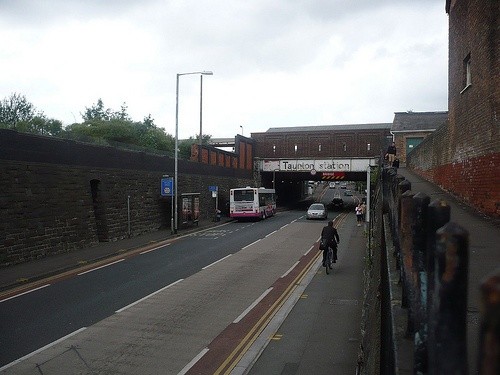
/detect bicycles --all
[320,238,339,275]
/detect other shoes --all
[322,261,326,267]
[357,224,361,226]
[332,260,336,263]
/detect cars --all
[340,184,346,189]
[305,202,328,221]
[329,182,335,189]
[332,197,343,207]
[344,190,353,196]
[335,181,340,184]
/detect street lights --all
[273,169,280,189]
[171,69,213,235]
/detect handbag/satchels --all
[319,239,329,250]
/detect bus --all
[227,186,277,223]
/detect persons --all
[361,202,366,222]
[355,204,362,224]
[387,142,396,167]
[216,210,221,222]
[321,221,339,267]
